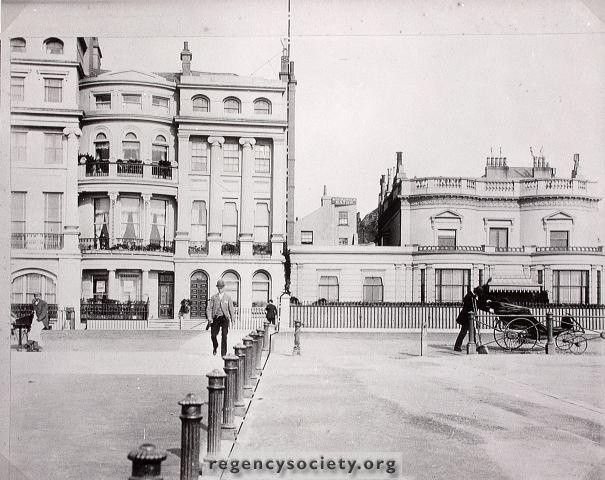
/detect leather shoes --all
[212,348,217,356]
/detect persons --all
[265,299,277,331]
[453,285,495,352]
[32,292,49,330]
[205,280,235,357]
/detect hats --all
[215,280,225,287]
